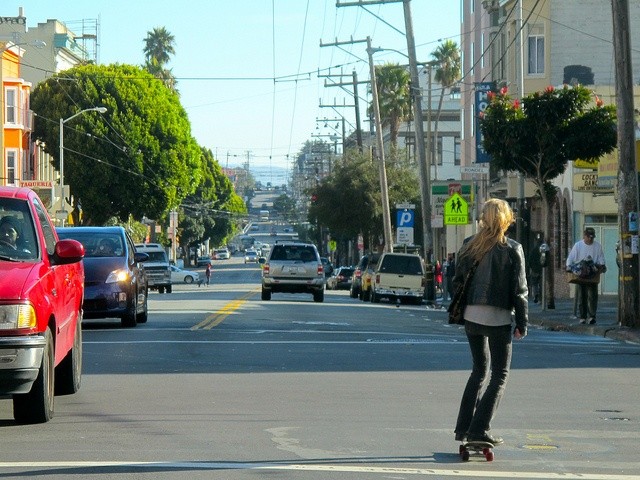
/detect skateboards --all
[459,437,494,461]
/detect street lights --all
[0,40,47,183]
[60,107,107,227]
[366,47,433,248]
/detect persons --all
[528,240,542,304]
[566,227,607,326]
[453,199,528,446]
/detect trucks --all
[259,210,269,221]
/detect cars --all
[282,227,294,232]
[262,204,268,209]
[261,243,325,302]
[326,268,339,289]
[360,253,381,301]
[226,244,238,255]
[321,257,333,276]
[270,229,278,237]
[332,267,355,290]
[350,256,369,298]
[256,186,280,191]
[250,224,259,231]
[54,226,149,327]
[244,251,258,264]
[248,240,277,258]
[216,249,230,260]
[197,256,211,266]
[171,265,200,284]
[370,253,426,305]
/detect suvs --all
[136,247,172,293]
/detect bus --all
[239,235,252,252]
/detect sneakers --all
[580,318,587,325]
[588,317,595,325]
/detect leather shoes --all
[454,430,469,440]
[466,431,504,446]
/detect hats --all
[584,227,596,238]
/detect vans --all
[134,243,161,248]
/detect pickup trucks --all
[0,184,85,423]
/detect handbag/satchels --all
[447,258,479,326]
